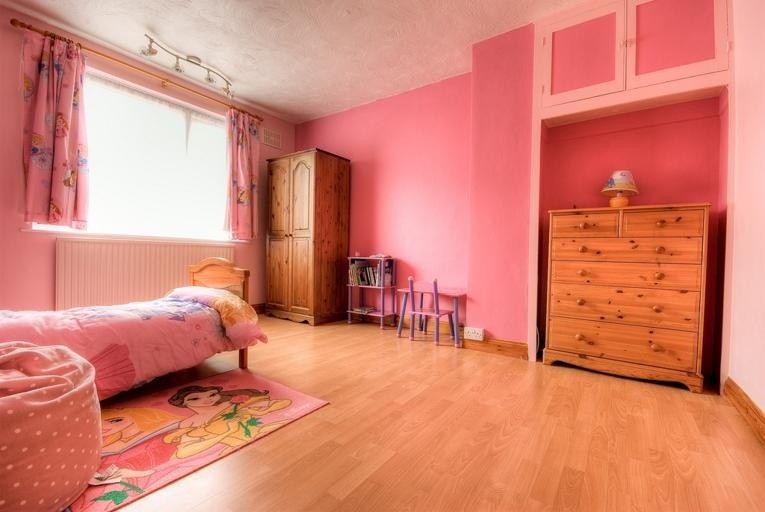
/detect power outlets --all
[463,327,485,342]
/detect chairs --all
[408,275,454,346]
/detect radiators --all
[55,236,235,310]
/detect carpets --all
[59,368,329,512]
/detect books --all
[352,306,374,315]
[349,260,393,286]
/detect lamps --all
[144,34,234,100]
[601,169,639,208]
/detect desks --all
[396,288,467,348]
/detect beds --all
[0,256,268,402]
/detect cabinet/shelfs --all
[543,201,712,394]
[540,0,730,130]
[346,256,398,331]
[265,147,350,326]
